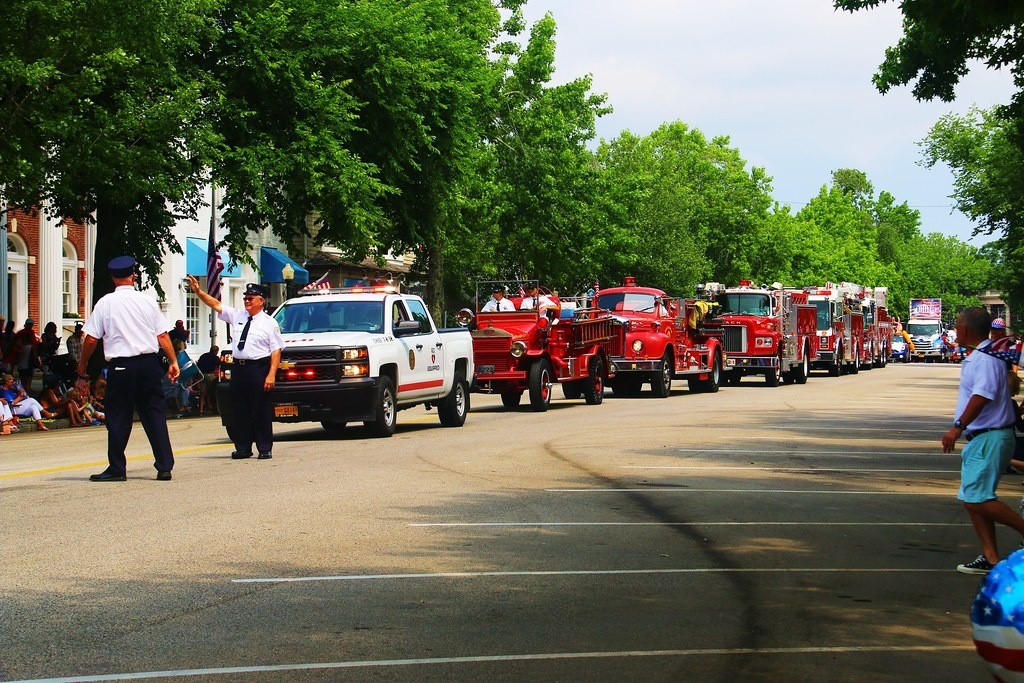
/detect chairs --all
[308,310,332,331]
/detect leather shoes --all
[157,472,171,479]
[258,451,273,459]
[90,471,126,481]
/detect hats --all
[176,320,182,326]
[25,318,34,324]
[491,285,506,292]
[528,282,537,289]
[244,283,265,296]
[108,256,135,275]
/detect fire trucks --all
[453,277,894,412]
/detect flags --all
[978,335,1024,367]
[206,221,225,302]
[303,277,332,289]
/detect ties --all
[497,302,500,311]
[533,298,536,306]
[238,315,253,350]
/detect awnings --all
[186,237,241,277]
[261,248,309,284]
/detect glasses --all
[243,297,254,301]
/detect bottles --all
[17,387,27,397]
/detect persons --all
[520,283,558,317]
[481,286,516,312]
[0,315,106,434]
[942,307,1024,574]
[189,275,286,460]
[78,257,180,482]
[162,320,220,418]
[990,318,1018,373]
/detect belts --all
[965,426,1011,441]
[233,358,264,366]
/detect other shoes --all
[39,426,49,431]
[46,412,58,420]
[71,423,81,427]
[82,423,91,426]
[12,426,20,431]
[1008,465,1024,475]
[173,412,184,418]
[199,411,206,417]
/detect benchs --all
[509,296,561,326]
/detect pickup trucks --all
[268,286,475,438]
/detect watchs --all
[954,420,966,430]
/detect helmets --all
[991,317,1006,329]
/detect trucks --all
[907,318,946,363]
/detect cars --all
[891,336,912,363]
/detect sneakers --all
[91,421,101,425]
[957,554,999,574]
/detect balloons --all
[969,548,1024,683]
